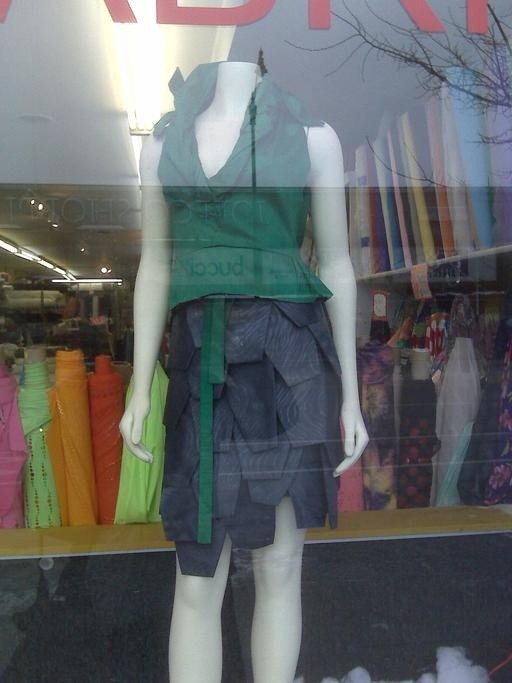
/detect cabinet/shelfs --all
[317,59,510,511]
